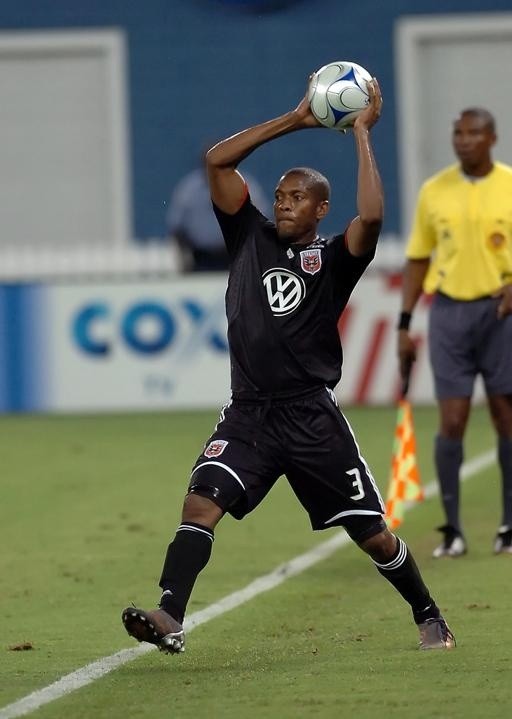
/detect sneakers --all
[415,613,458,653]
[491,523,512,556]
[431,521,469,560]
[120,602,187,657]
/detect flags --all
[380,399,425,532]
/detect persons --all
[122,62,452,660]
[394,106,512,556]
[163,136,271,271]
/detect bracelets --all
[396,311,411,330]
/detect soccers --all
[309,61,375,129]
